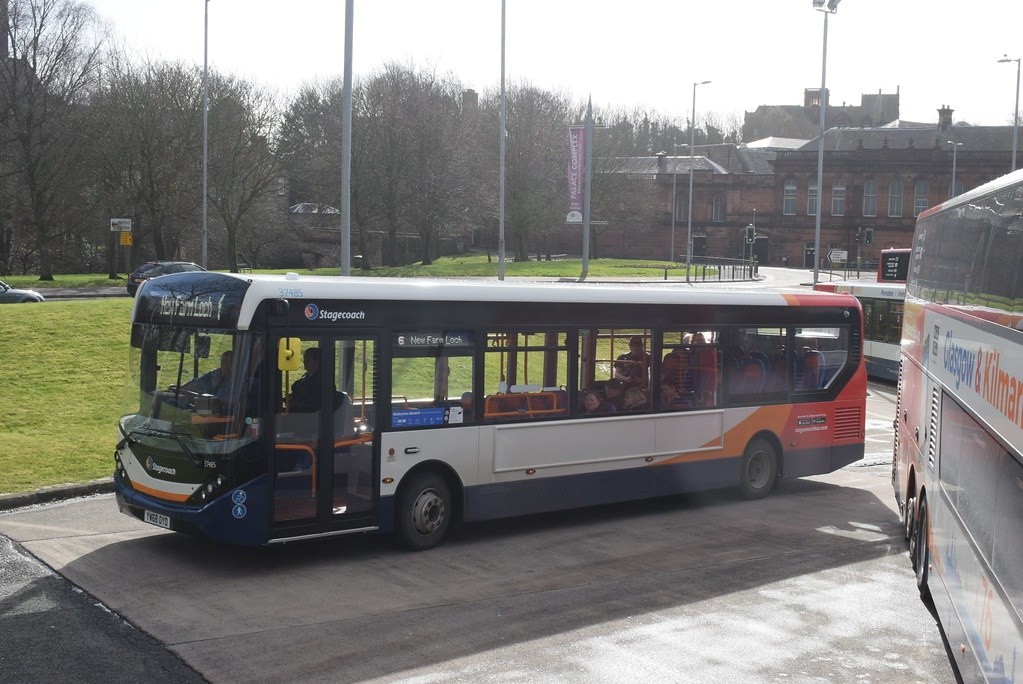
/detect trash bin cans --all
[806,249,815,267]
[354,255,362,268]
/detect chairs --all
[332,346,822,449]
[392,408,445,428]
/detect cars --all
[331,254,363,269]
[0,280,46,308]
[289,202,341,215]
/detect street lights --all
[947,141,966,199]
[684,81,713,282]
[811,0,842,285]
[998,57,1021,173]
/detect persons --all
[167,350,233,410]
[613,335,650,389]
[660,333,717,409]
[582,392,607,414]
[283,348,322,413]
[623,386,649,410]
[595,378,625,412]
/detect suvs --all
[128,260,208,298]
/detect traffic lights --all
[865,228,875,245]
[856,231,863,244]
[746,226,757,244]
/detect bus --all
[885,169,1022,683]
[877,246,915,286]
[813,281,912,390]
[110,269,866,559]
